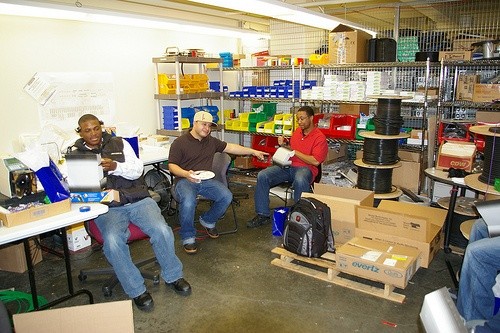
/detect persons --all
[64,114,192,311]
[168,110,270,254]
[246,106,328,229]
[455,218,500,333]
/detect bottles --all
[336,37,347,64]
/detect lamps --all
[0,0,377,37]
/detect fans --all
[145,172,176,217]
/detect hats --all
[193,111,218,127]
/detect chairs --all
[267,159,322,210]
[77,215,160,295]
[192,153,240,239]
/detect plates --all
[191,171,214,180]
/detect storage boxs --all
[157,23,500,290]
[0,237,43,273]
[12,299,135,333]
[70,189,121,203]
[67,224,93,252]
[0,196,73,227]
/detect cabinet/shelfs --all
[295,56,444,189]
[436,59,500,174]
[208,60,302,199]
[152,56,223,137]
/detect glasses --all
[296,115,309,122]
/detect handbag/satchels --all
[271,207,288,237]
[29,151,72,204]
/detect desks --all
[424,168,479,254]
[0,202,111,310]
[138,133,181,165]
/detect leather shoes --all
[133,290,154,312]
[165,278,192,296]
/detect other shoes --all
[205,226,220,239]
[183,243,197,254]
[246,214,272,227]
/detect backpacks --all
[283,197,336,258]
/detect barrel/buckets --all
[482,42,494,58]
[398,195,432,207]
